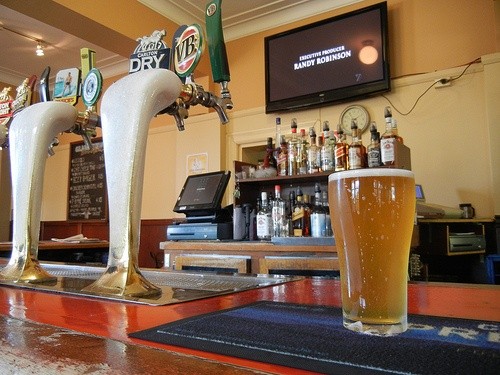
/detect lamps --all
[35,40,46,57]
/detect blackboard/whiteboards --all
[66,136,108,221]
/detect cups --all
[459,204,475,219]
[328,168,416,334]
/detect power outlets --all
[433,76,452,88]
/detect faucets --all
[179,0,234,124]
[153,22,205,131]
[65,124,98,151]
[36,65,60,156]
[74,67,103,129]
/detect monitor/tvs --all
[263,0,391,114]
[173,170,231,222]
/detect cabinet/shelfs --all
[233,139,411,209]
[416,217,495,258]
[172,255,342,280]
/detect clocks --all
[339,104,370,137]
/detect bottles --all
[243,182,333,240]
[257,106,404,175]
